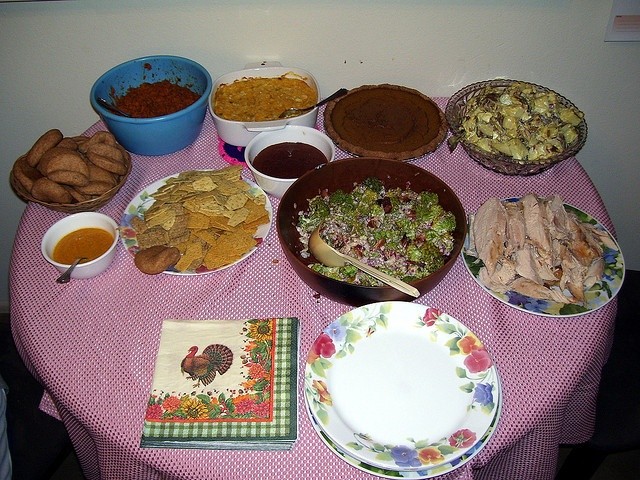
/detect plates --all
[303,301,503,480]
[461,196,626,317]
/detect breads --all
[134,244,181,276]
[12,128,128,204]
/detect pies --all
[323,84,451,162]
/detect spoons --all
[307,222,421,299]
[279,87,348,120]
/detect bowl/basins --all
[90,55,213,156]
[275,157,468,308]
[208,60,321,148]
[243,124,336,199]
[40,211,120,280]
[444,78,588,178]
